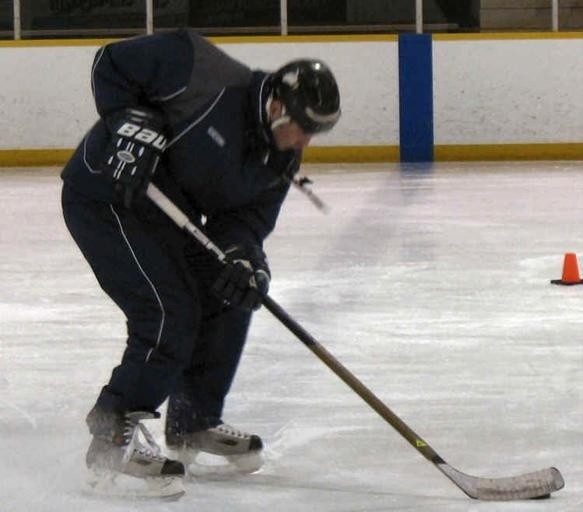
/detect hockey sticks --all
[147,182,564,501]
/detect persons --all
[57,31,340,499]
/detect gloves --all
[103,107,167,210]
[207,240,270,312]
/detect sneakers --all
[85,408,185,478]
[165,414,264,456]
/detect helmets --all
[269,58,341,133]
